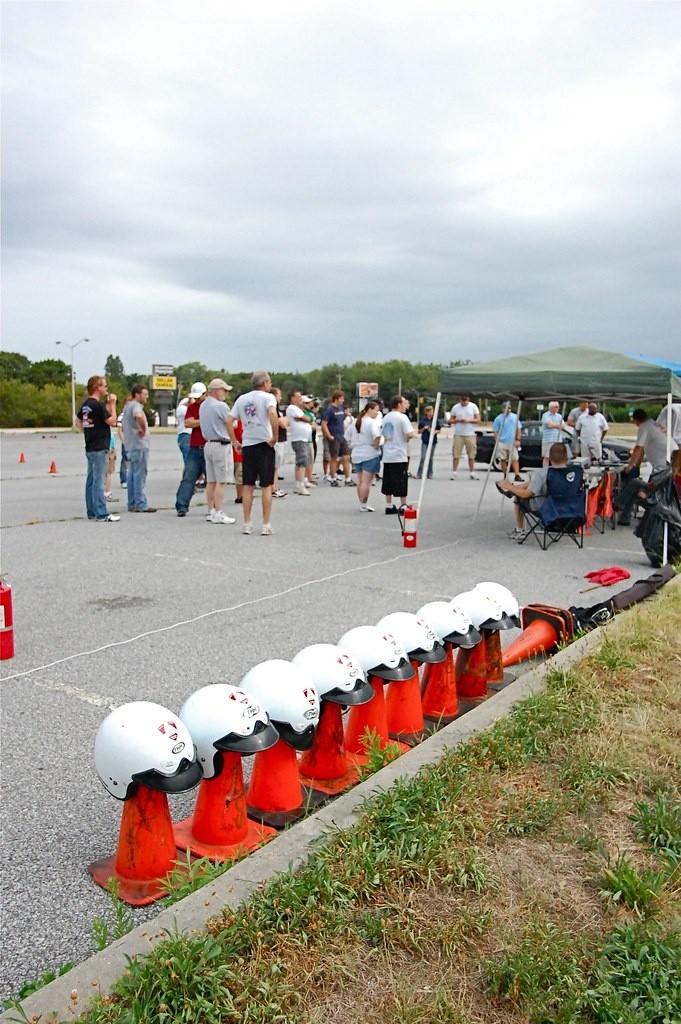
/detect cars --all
[474,420,647,472]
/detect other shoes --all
[506,528,526,539]
[450,473,456,480]
[515,475,524,481]
[495,481,515,499]
[469,475,479,481]
[235,467,432,515]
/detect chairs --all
[513,464,588,551]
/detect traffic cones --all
[171,740,278,865]
[343,671,410,765]
[88,773,211,907]
[294,696,379,796]
[502,603,573,667]
[383,659,445,748]
[421,637,475,725]
[244,722,331,832]
[454,636,497,708]
[480,628,515,693]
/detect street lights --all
[47,460,57,473]
[56,338,90,428]
[17,453,26,463]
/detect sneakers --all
[96,483,205,517]
[97,514,122,521]
[262,525,275,535]
[242,524,254,535]
[207,511,235,524]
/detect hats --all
[208,379,233,391]
[300,395,313,404]
[188,382,206,399]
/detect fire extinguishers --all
[0,573,14,661]
[398,503,417,547]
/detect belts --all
[206,440,220,442]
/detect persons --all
[575,403,609,460]
[541,401,565,468]
[285,389,313,496]
[224,370,279,535]
[449,395,481,481]
[175,382,208,517]
[382,395,415,516]
[492,402,525,482]
[414,405,442,479]
[199,378,236,524]
[122,382,158,512]
[75,375,121,522]
[343,401,382,512]
[321,389,358,487]
[624,408,681,520]
[495,443,569,540]
[75,370,681,540]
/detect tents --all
[417,345,681,520]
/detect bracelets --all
[231,440,239,446]
[516,439,520,442]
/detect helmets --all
[380,612,447,663]
[294,643,374,718]
[93,702,205,801]
[454,589,516,630]
[179,683,279,780]
[339,626,416,685]
[474,580,523,629]
[417,600,482,649]
[239,659,321,751]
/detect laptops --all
[603,447,629,464]
[588,446,625,466]
